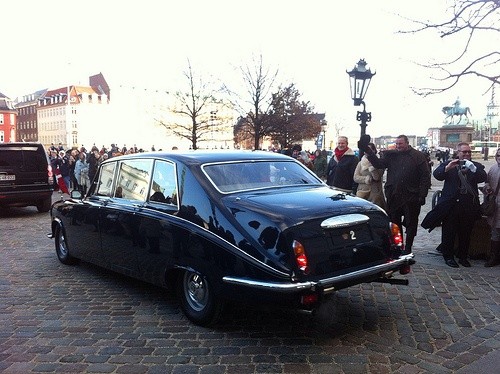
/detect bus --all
[469,140,500,157]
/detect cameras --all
[457,160,466,166]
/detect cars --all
[46,149,417,327]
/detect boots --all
[484,240,500,267]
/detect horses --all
[441,106,472,125]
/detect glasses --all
[496,155,500,157]
[459,150,471,154]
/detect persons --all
[279,134,500,267]
[48,143,178,196]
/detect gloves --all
[462,159,476,173]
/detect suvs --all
[0,141,55,212]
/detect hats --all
[291,144,302,151]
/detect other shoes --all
[458,258,470,267]
[446,258,458,267]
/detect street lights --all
[346,57,377,135]
